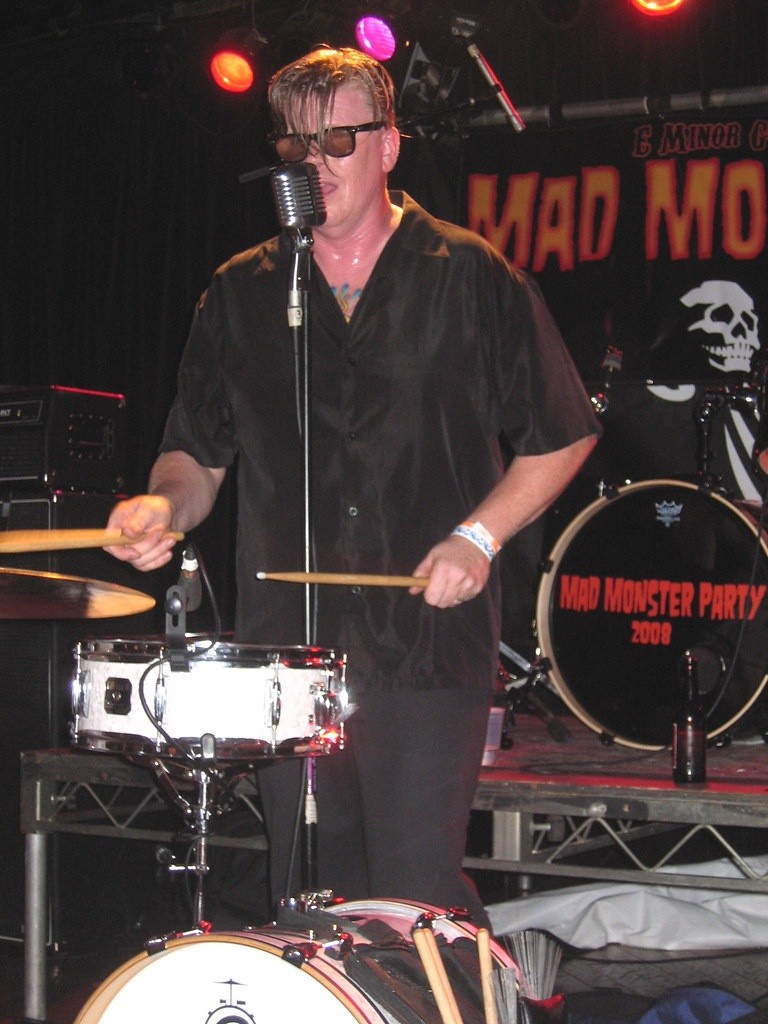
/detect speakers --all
[1,491,141,952]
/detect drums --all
[74,621,361,771]
[534,472,768,755]
[73,899,548,1024]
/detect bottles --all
[671,651,706,784]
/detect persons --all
[101,44,602,942]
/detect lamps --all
[207,26,272,96]
[352,0,418,65]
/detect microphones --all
[271,161,331,331]
[180,543,203,612]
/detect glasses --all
[264,120,388,164]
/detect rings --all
[457,594,464,601]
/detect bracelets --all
[450,522,503,562]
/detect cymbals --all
[0,565,159,619]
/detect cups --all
[480,708,506,766]
